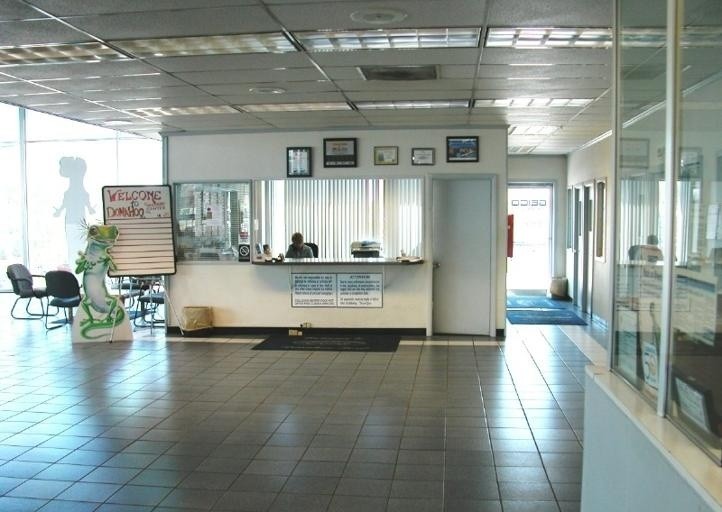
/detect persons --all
[633,235,662,261]
[263,244,272,256]
[285,232,313,258]
[650,303,660,356]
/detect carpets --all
[251,332,400,352]
[506,294,568,310]
[508,309,588,327]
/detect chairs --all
[8,263,168,328]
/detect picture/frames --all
[446,136,480,163]
[620,137,650,169]
[323,138,358,168]
[411,148,436,167]
[373,146,400,167]
[286,146,313,177]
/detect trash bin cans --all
[550,277,568,299]
[183,306,213,337]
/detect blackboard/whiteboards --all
[102,184,178,278]
